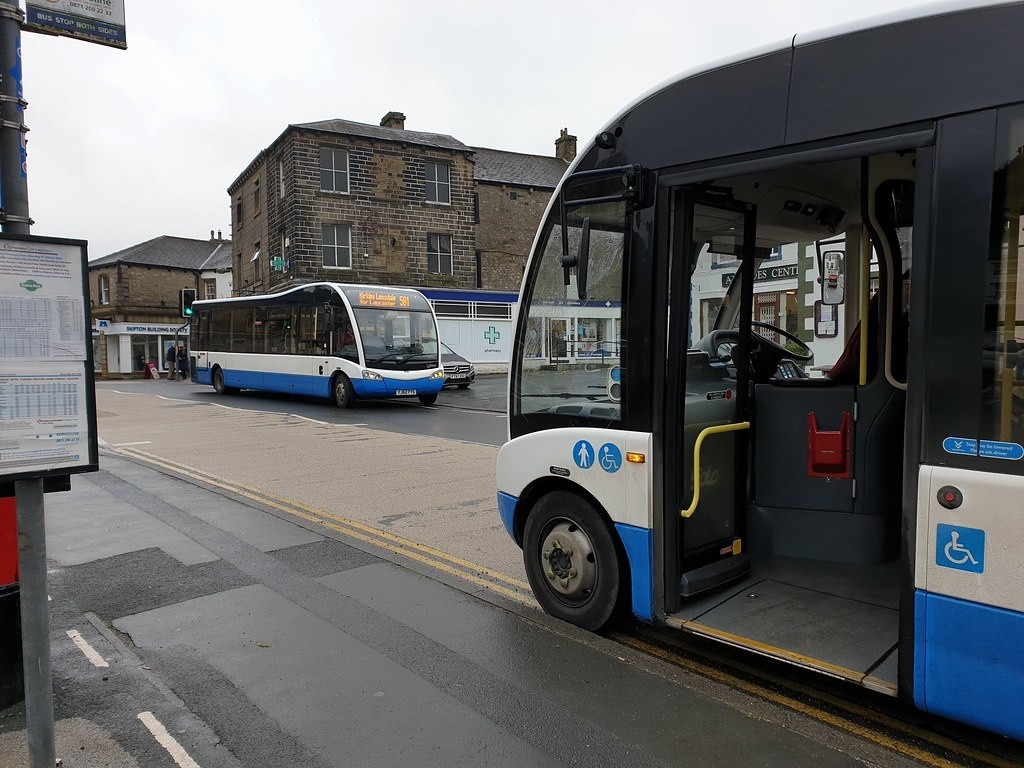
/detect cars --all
[382,336,476,392]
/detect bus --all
[498,2,1024,746]
[190,279,445,406]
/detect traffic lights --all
[179,288,196,318]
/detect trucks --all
[827,274,838,287]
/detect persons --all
[165,343,178,381]
[342,320,357,347]
[176,344,189,380]
